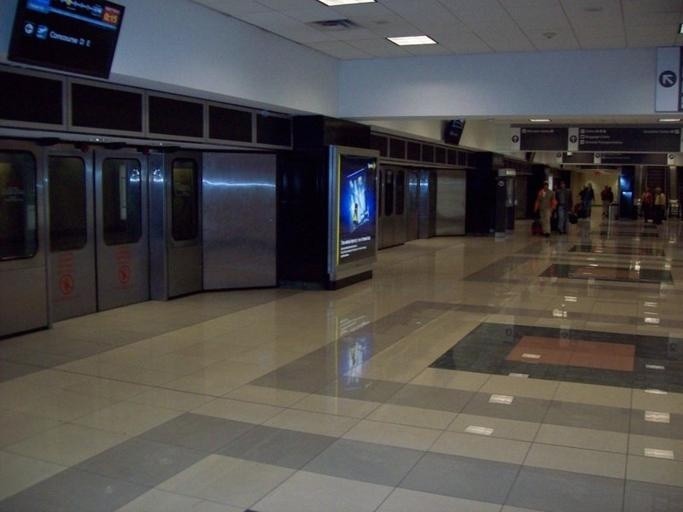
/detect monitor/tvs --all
[8,0,125,78]
[445,119,465,144]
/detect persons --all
[532,180,667,238]
